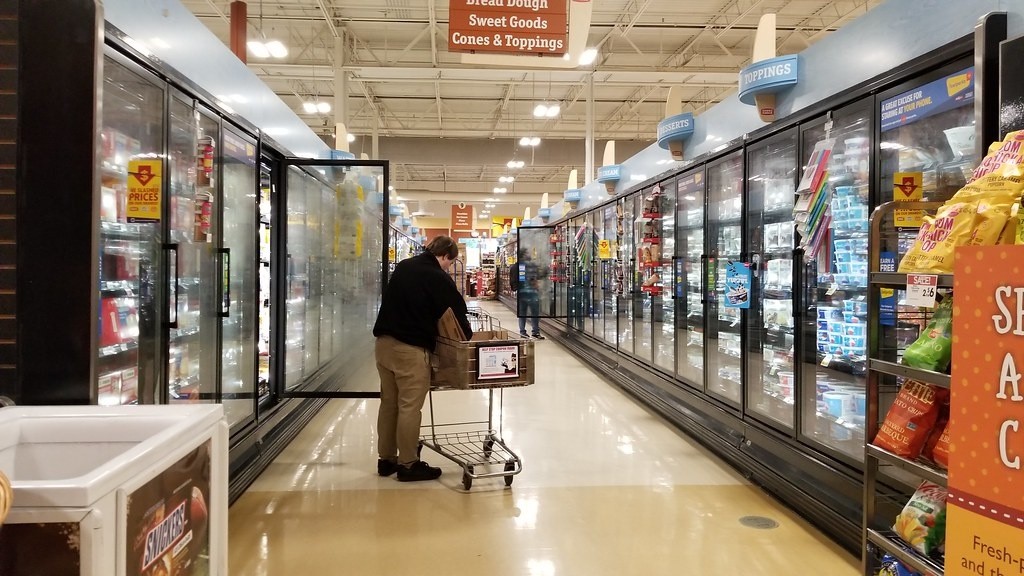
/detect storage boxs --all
[482,269,496,286]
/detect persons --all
[509,247,550,340]
[372,236,473,482]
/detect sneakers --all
[520,330,534,339]
[531,330,545,340]
[377,458,397,476]
[397,461,443,482]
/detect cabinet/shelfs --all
[480,263,496,301]
[861,200,953,576]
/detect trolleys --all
[416,307,536,491]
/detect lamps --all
[519,119,541,148]
[247,0,288,59]
[302,27,332,114]
[533,72,562,118]
